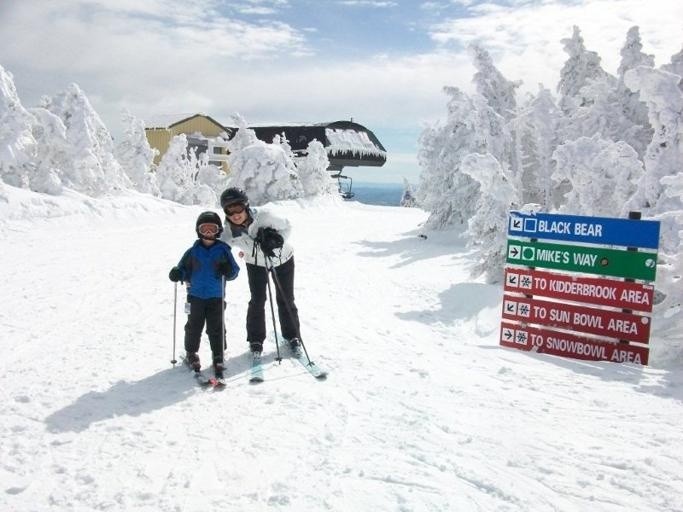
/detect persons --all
[168,211,240,373]
[217,187,303,361]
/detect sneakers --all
[214,356,224,371]
[250,341,263,352]
[187,352,201,369]
[289,337,301,353]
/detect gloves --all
[218,257,232,274]
[256,227,284,257]
[169,268,180,282]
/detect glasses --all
[197,223,220,236]
[224,200,250,216]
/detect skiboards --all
[180,353,226,387]
[250,339,329,383]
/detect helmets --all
[220,187,249,208]
[196,212,222,238]
